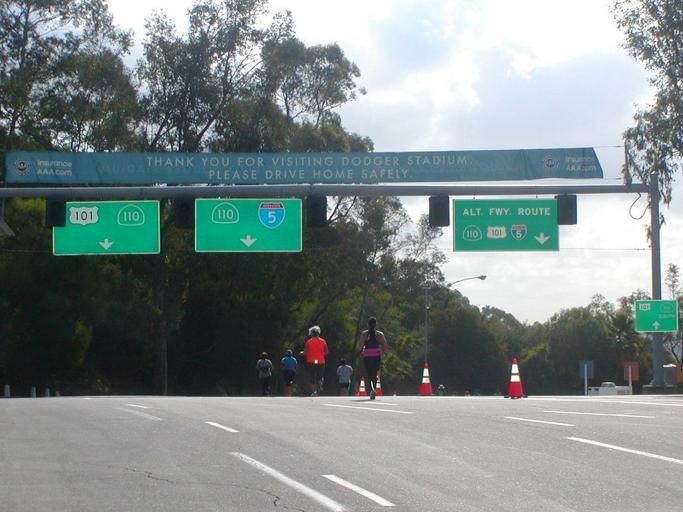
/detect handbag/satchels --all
[259,366,269,373]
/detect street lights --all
[425,276,487,363]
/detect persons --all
[357,315,389,400]
[464,383,471,396]
[256,351,274,396]
[299,325,330,396]
[336,358,354,395]
[280,350,298,396]
[436,384,446,395]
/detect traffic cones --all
[419,362,432,395]
[356,377,368,396]
[505,358,527,399]
[373,376,382,396]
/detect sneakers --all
[310,377,324,397]
[370,391,375,400]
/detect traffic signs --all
[635,300,678,333]
[453,199,558,251]
